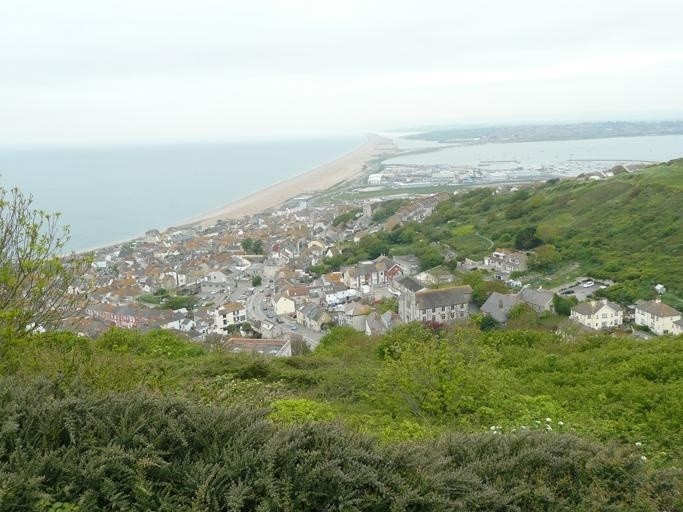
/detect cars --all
[559,289,574,294]
[582,281,594,288]
[191,279,297,330]
